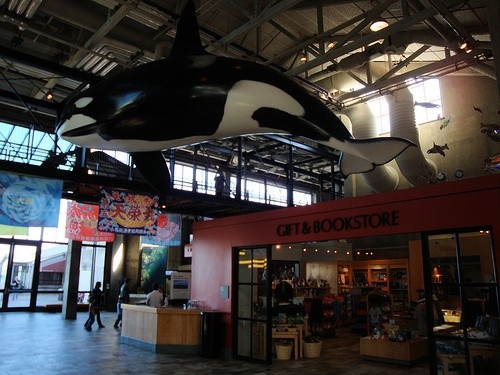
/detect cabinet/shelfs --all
[336,260,462,365]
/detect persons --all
[11,276,22,300]
[84,279,169,333]
[215,173,224,196]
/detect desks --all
[259,314,309,360]
[121,303,211,355]
[437,328,500,375]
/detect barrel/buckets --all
[304,343,320,358]
[276,345,293,361]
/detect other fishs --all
[414,100,440,109]
[426,142,449,157]
[440,117,451,130]
[473,106,482,113]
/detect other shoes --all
[86,327,91,331]
[114,324,119,328]
[99,325,105,328]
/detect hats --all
[124,278,131,281]
